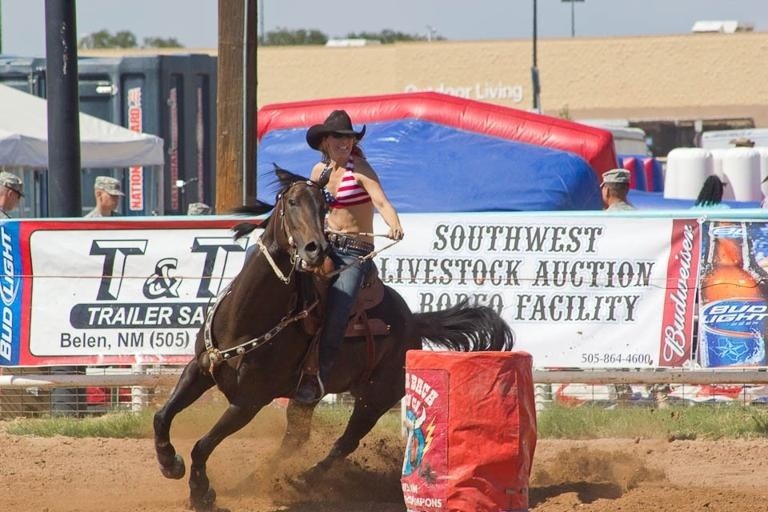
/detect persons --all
[0,171,25,220]
[80,174,126,217]
[290,109,406,407]
[598,168,637,211]
[689,173,731,210]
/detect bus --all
[626,117,759,164]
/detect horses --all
[153,164,518,512]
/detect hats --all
[0,171,24,197]
[95,176,125,196]
[306,110,366,151]
[188,203,209,214]
[599,169,631,187]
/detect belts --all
[324,232,373,252]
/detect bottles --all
[696,221,768,391]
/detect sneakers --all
[296,374,328,400]
